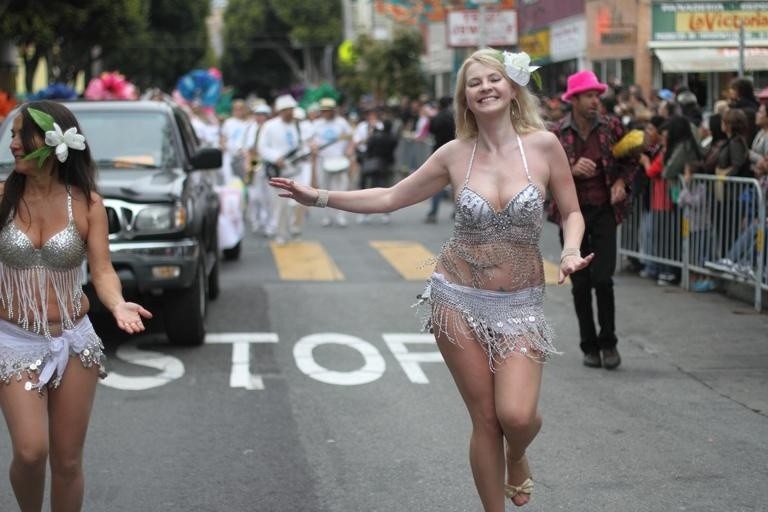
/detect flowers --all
[497,49,543,92]
[23,107,86,167]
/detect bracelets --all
[559,248,580,262]
[314,189,330,208]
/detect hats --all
[560,70,608,104]
[250,93,339,119]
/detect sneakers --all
[733,262,752,278]
[619,256,719,292]
[702,257,733,273]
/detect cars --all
[190,119,248,262]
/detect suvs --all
[1,98,235,355]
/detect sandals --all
[503,440,534,506]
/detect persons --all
[268,46,595,512]
[546,69,639,369]
[187,92,456,239]
[0,102,153,511]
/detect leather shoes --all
[602,341,622,370]
[583,347,602,368]
